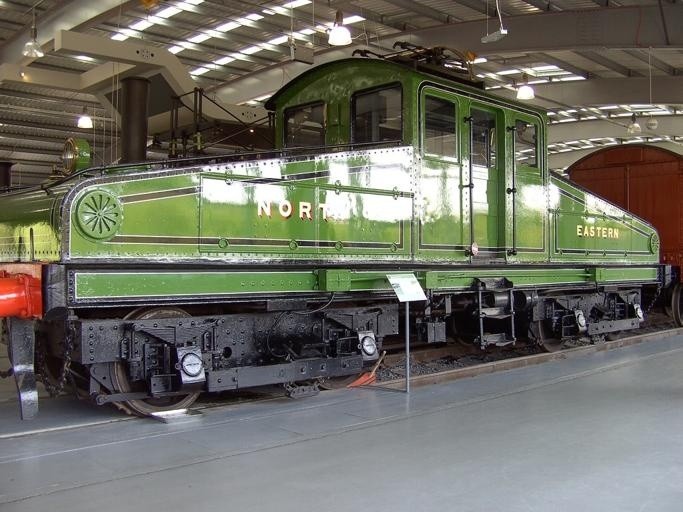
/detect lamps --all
[22,7,44,58]
[328,9,352,46]
[628,112,640,133]
[516,73,535,100]
[77,103,93,129]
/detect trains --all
[0,38,683,421]
